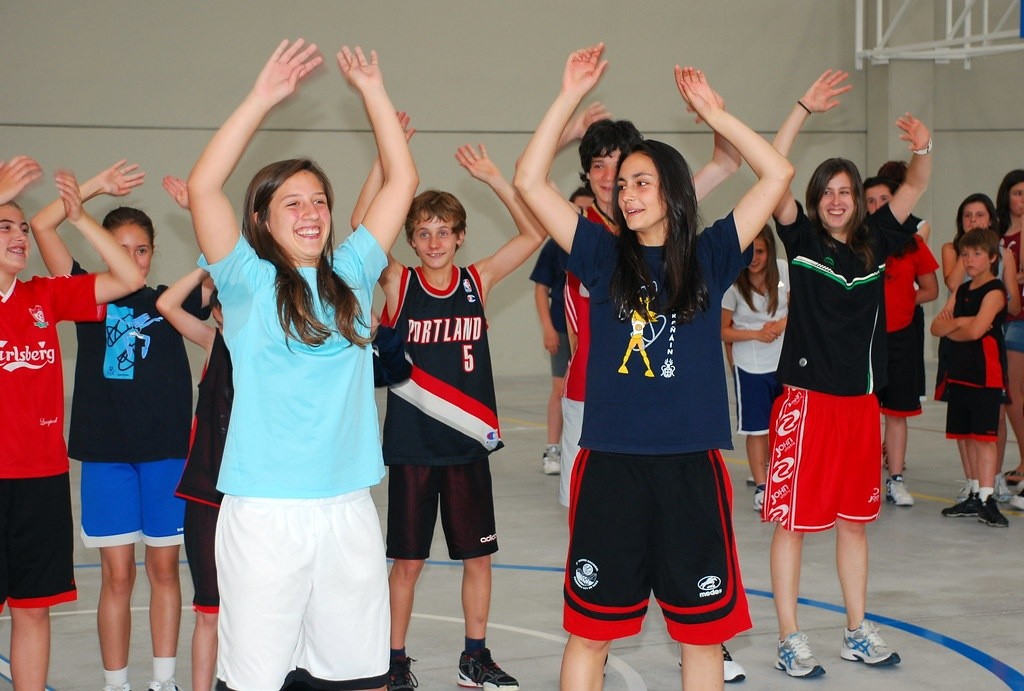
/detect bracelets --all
[797,101,812,114]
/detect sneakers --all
[886,474,913,505]
[840,621,901,666]
[457,647,520,691]
[956,482,970,502]
[679,643,745,682]
[753,489,766,511]
[881,443,905,469]
[388,655,419,691]
[942,493,982,517]
[992,475,1012,502]
[1010,478,1023,510]
[774,633,826,677]
[978,495,1008,526]
[542,446,560,475]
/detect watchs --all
[913,138,933,154]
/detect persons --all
[771,66,934,679]
[0,155,146,691]
[863,160,1024,528]
[28,159,216,691]
[156,37,419,691]
[513,41,796,691]
[351,111,548,691]
